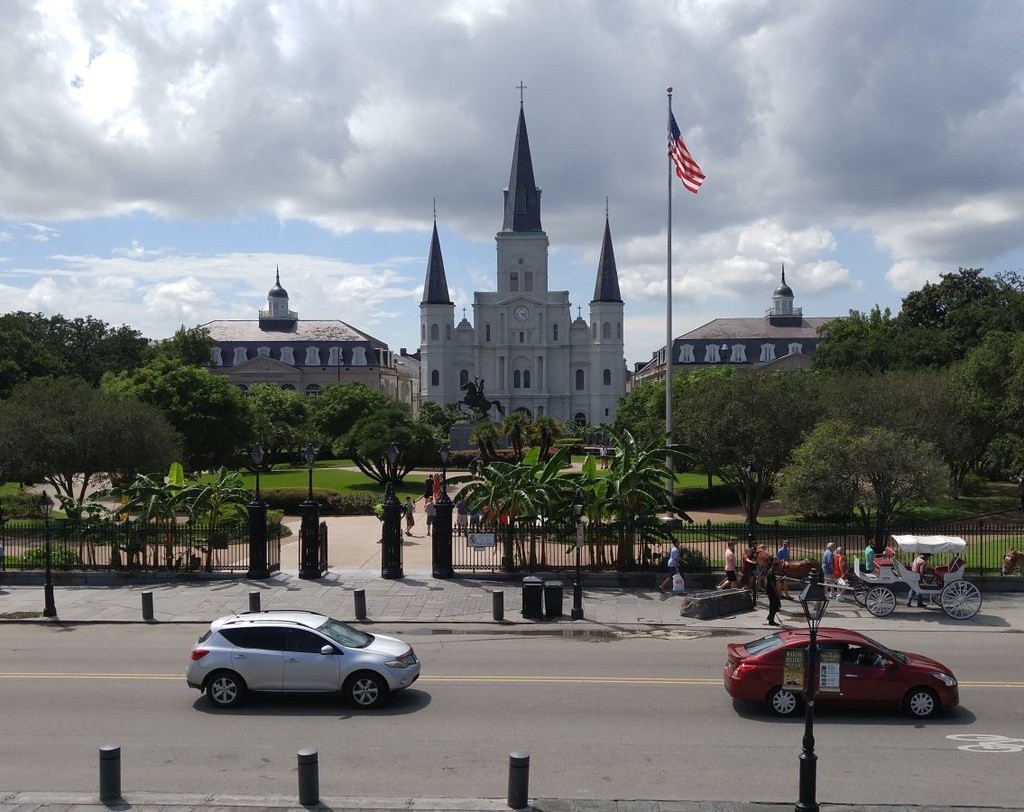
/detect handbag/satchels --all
[672,568,684,591]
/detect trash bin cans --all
[522,576,543,619]
[544,581,564,616]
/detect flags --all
[664,110,706,194]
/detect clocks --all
[513,304,531,322]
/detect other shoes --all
[918,603,927,607]
[717,586,722,591]
[715,584,718,587]
[830,591,838,598]
[769,619,780,626]
[906,602,912,607]
[778,594,785,600]
[767,616,770,619]
[836,594,845,602]
[786,595,795,600]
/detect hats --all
[869,539,877,547]
[923,553,934,557]
[784,541,790,545]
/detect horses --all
[750,549,821,601]
[1003,550,1024,577]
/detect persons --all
[766,562,782,626]
[455,497,510,537]
[907,551,933,608]
[864,538,877,573]
[833,547,848,602]
[822,542,835,599]
[424,496,436,536]
[598,444,608,469]
[466,378,484,408]
[657,539,690,596]
[424,474,434,507]
[433,473,442,501]
[715,535,795,600]
[403,496,416,536]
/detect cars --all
[724,627,959,718]
[186,610,421,709]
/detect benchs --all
[892,557,921,582]
[873,556,893,578]
[935,555,965,582]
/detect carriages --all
[754,534,982,620]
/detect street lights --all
[794,569,830,812]
[570,489,584,620]
[706,518,711,573]
[38,489,57,617]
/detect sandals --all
[660,585,666,593]
[679,590,688,594]
